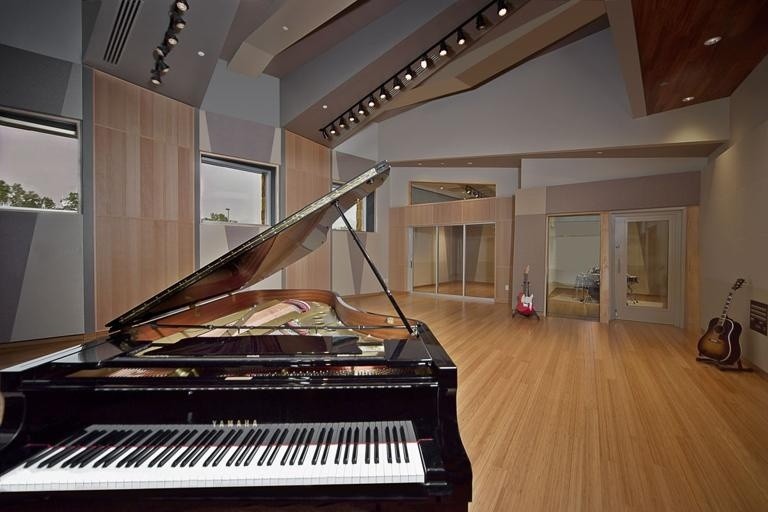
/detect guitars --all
[515,266,535,315]
[697,279,748,364]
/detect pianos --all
[0,160,472,511]
